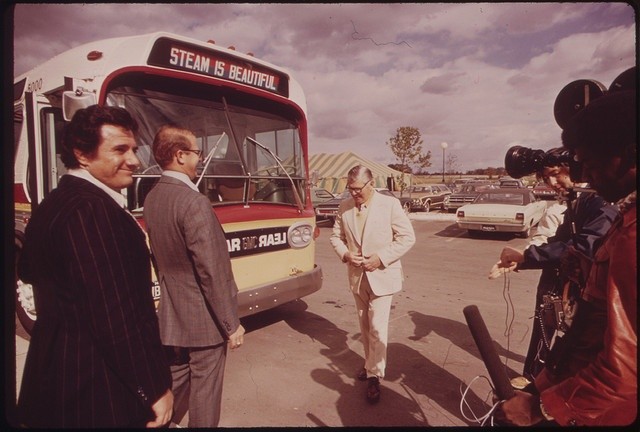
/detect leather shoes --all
[357,368,367,381]
[366,376,381,402]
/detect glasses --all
[345,179,371,192]
[182,148,203,158]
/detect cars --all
[443,180,485,212]
[533,181,559,200]
[315,188,412,224]
[401,183,452,211]
[455,179,548,237]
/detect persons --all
[489,202,574,390]
[495,92,637,426]
[500,148,622,365]
[15,104,177,428]
[143,125,247,428]
[330,165,418,404]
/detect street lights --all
[441,142,447,181]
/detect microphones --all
[463,305,515,400]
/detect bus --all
[12,30,322,337]
